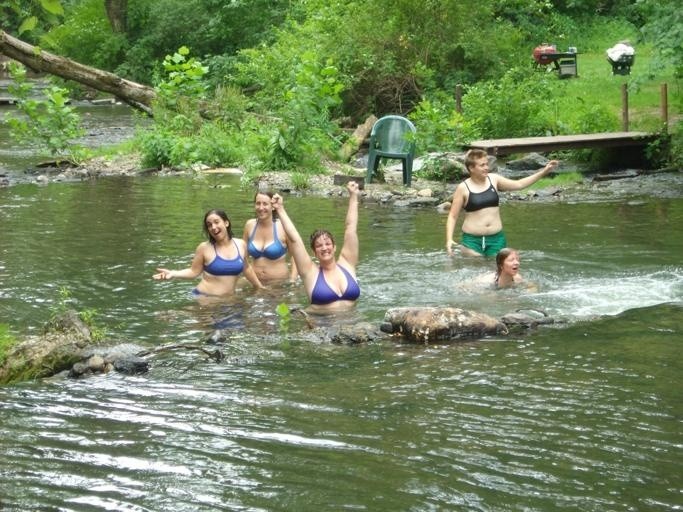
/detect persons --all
[242,186,297,287]
[481,247,537,292]
[445,148,559,256]
[270,180,361,317]
[152,208,268,296]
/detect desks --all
[541,52,585,77]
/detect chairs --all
[366,115,417,188]
